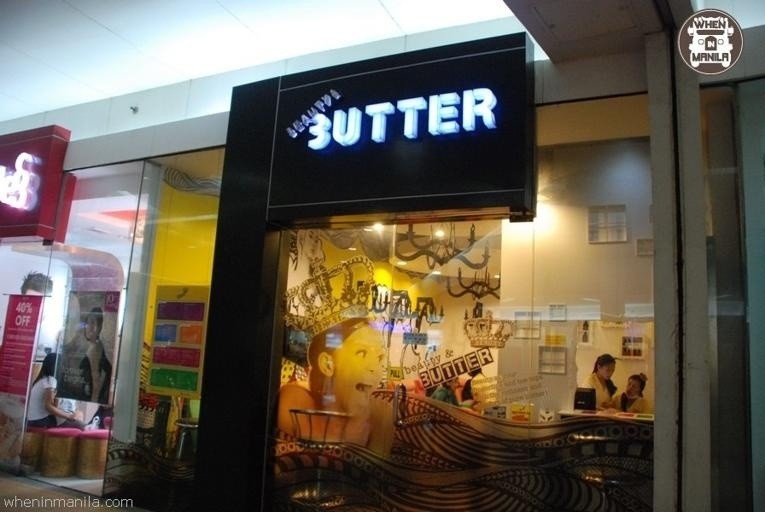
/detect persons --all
[429,376,474,408]
[27,355,85,432]
[612,374,651,414]
[276,316,394,462]
[582,354,615,409]
[79,307,112,405]
[461,366,487,402]
[20,271,53,324]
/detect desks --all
[290,408,350,508]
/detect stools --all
[77,430,109,479]
[25,425,46,468]
[42,427,81,477]
